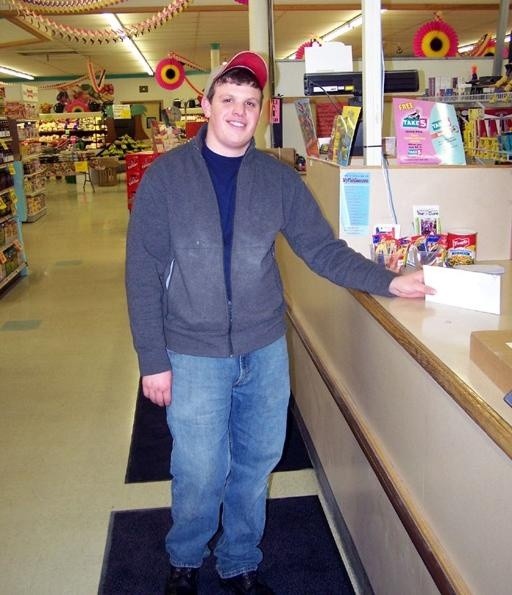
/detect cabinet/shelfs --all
[0,102,209,289]
[415,92,512,163]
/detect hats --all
[203,50,267,98]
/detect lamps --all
[283,8,388,60]
[104,12,153,76]
[457,33,510,54]
[0,66,34,80]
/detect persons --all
[124,50,436,594]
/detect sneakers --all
[167,566,198,595]
[218,570,275,595]
